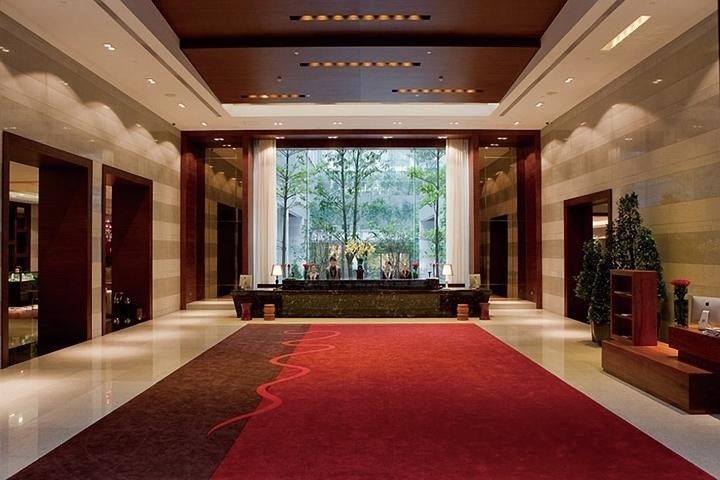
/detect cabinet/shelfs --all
[600,336,710,416]
[610,270,657,346]
[667,325,719,412]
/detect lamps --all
[441,264,453,290]
[270,263,282,288]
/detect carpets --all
[7,322,717,480]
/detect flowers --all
[345,239,375,259]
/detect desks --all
[440,287,492,320]
[232,288,282,317]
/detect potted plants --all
[576,239,611,345]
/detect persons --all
[306,263,320,280]
[380,260,396,279]
[325,255,342,279]
[398,261,412,279]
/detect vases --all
[355,259,364,278]
